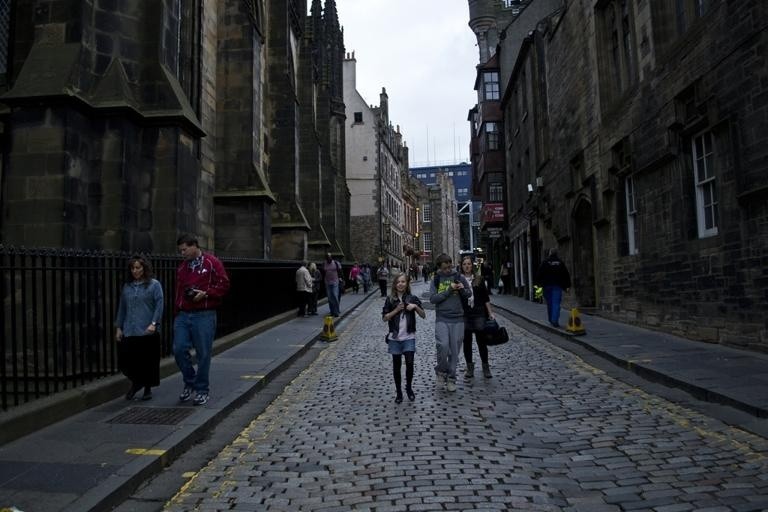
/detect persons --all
[297,247,513,318]
[456,253,496,379]
[381,271,426,403]
[114,253,165,400]
[429,252,472,391]
[538,247,574,327]
[170,232,232,406]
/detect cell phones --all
[454,280,458,284]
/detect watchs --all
[151,321,158,325]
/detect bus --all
[455,249,474,273]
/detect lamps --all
[527,176,543,198]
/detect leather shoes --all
[406,390,415,402]
[395,392,403,404]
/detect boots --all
[464,361,475,378]
[481,362,492,378]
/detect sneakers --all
[330,313,339,317]
[179,383,194,402]
[192,390,210,405]
[140,388,153,401]
[307,312,319,316]
[125,386,139,400]
[435,371,448,391]
[553,322,560,328]
[446,374,458,392]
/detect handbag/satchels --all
[476,316,509,347]
[385,333,390,344]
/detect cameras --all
[184,288,196,300]
